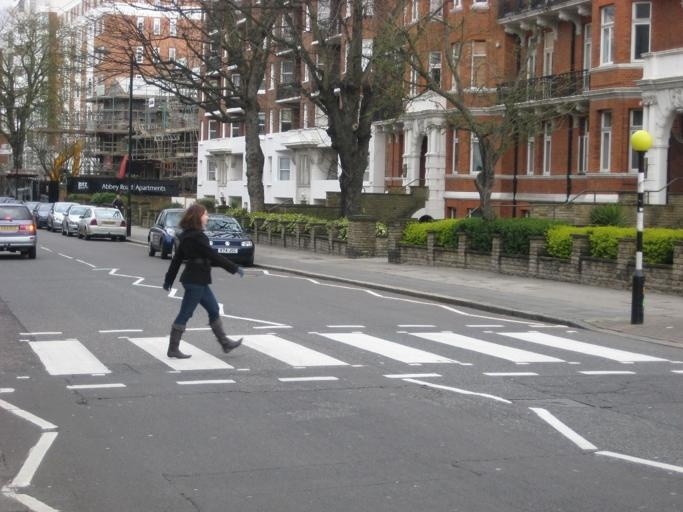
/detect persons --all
[160,202,248,360]
[112,194,125,213]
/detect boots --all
[165,323,192,360]
[208,316,244,355]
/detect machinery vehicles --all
[50,140,85,182]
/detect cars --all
[171,213,255,267]
[0,197,54,229]
[77,207,128,241]
[46,201,80,232]
[0,204,38,259]
[62,205,96,236]
[148,208,187,259]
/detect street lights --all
[631,129,653,325]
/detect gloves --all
[161,282,171,292]
[236,268,244,277]
